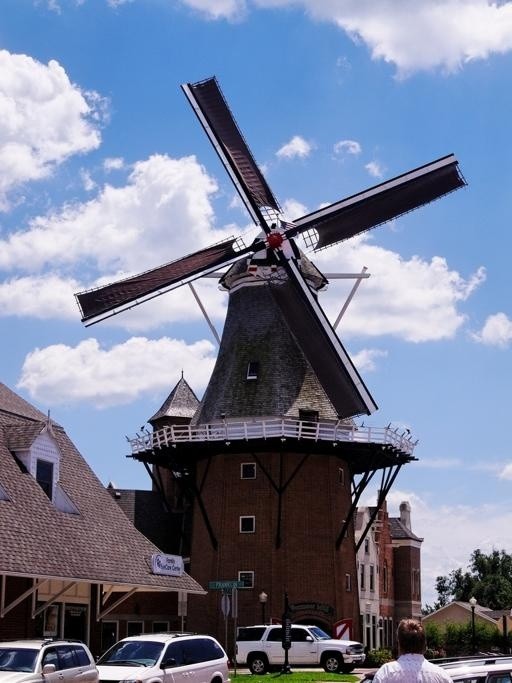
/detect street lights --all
[469,594,479,651]
[258,590,269,624]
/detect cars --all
[358,655,511,682]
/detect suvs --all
[235,623,367,674]
[0,639,100,683]
[93,630,233,683]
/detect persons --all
[370,618,456,683]
[432,645,446,663]
